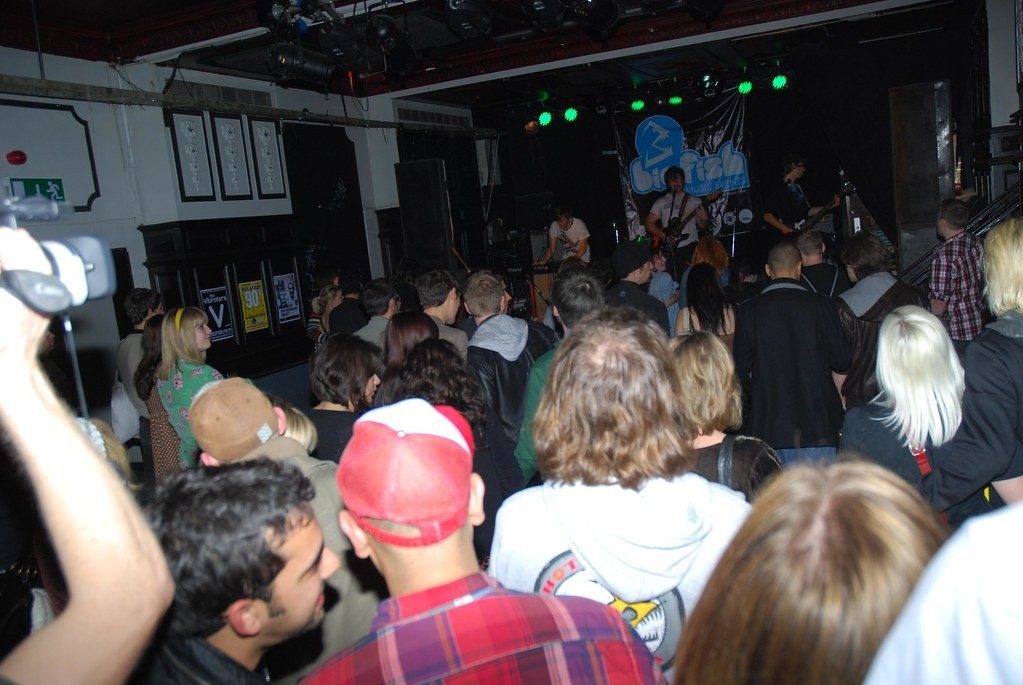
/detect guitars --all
[798,181,856,231]
[659,189,722,250]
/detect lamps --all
[665,87,684,107]
[264,41,335,92]
[526,0,563,39]
[768,68,788,92]
[686,0,726,29]
[520,95,645,136]
[365,16,420,81]
[573,0,622,42]
[445,1,495,48]
[736,73,752,95]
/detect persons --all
[486,305,753,684]
[837,304,1006,536]
[125,455,342,684]
[667,331,786,505]
[0,152,1022,684]
[292,397,670,684]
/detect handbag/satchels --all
[111,340,140,444]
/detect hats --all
[187,376,277,462]
[337,396,474,546]
[613,243,652,278]
[782,153,808,164]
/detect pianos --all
[504,263,591,311]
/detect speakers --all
[394,158,459,273]
[508,265,559,324]
[890,76,981,226]
[897,224,941,287]
[511,231,565,268]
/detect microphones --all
[672,183,679,193]
[787,178,792,190]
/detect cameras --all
[38,233,117,308]
